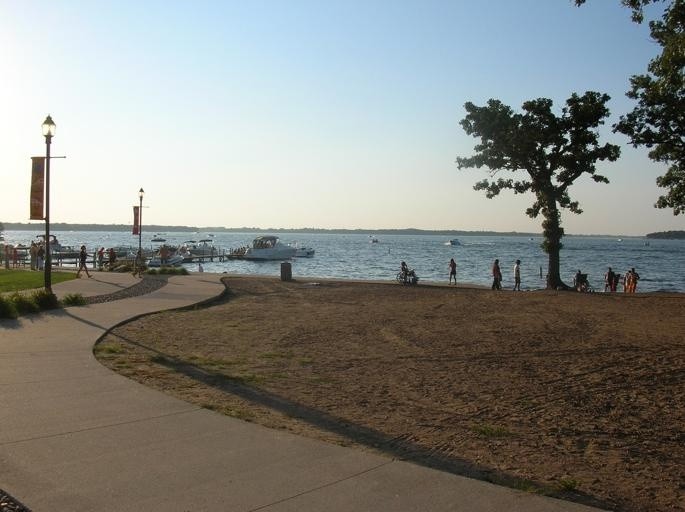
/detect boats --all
[35,232,79,258]
[106,239,217,267]
[449,239,462,246]
[243,235,299,260]
[296,248,314,258]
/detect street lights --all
[40,115,55,289]
[136,188,145,257]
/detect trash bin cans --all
[280,263,292,282]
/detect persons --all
[401,261,420,280]
[96,246,105,270]
[448,258,457,286]
[491,259,501,290]
[604,267,640,292]
[4,239,46,270]
[131,250,143,278]
[76,244,95,278]
[108,248,116,267]
[159,243,168,266]
[576,269,582,274]
[512,260,521,291]
